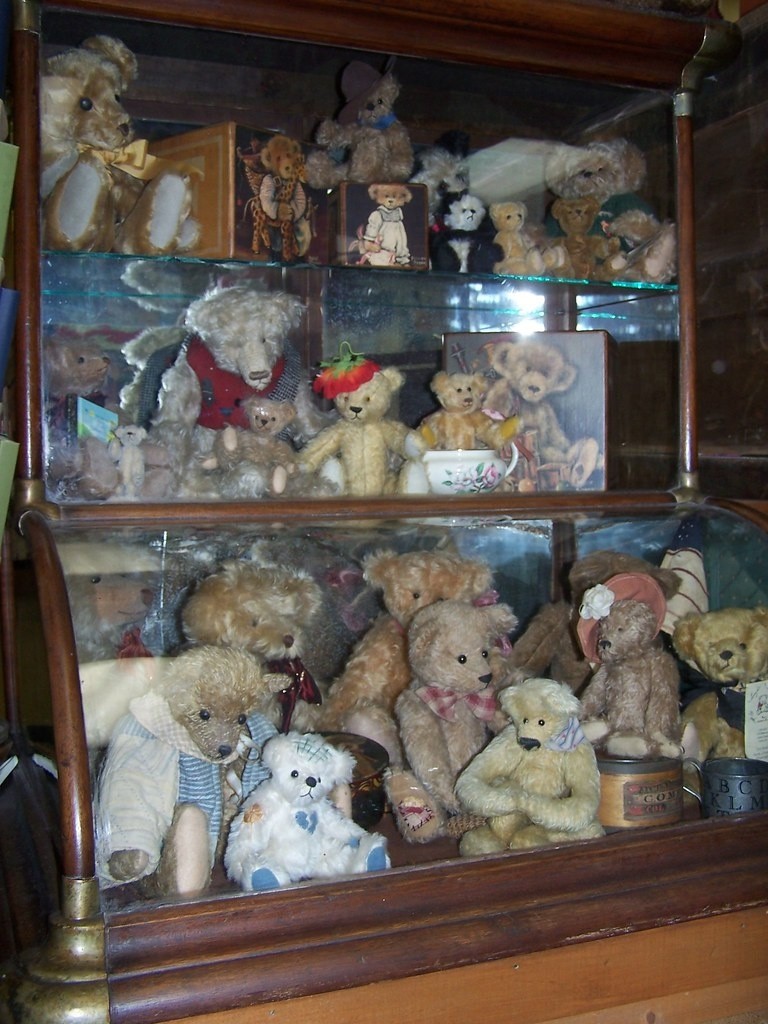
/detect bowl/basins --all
[422,439,518,495]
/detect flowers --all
[312,340,381,399]
[579,582,616,621]
[442,461,500,494]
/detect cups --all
[682,757,768,819]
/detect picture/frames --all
[441,329,610,495]
[66,392,120,450]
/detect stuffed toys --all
[33,34,768,896]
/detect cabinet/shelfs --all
[1,0,767,1024]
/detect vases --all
[680,757,768,820]
[419,441,517,496]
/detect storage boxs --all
[111,122,329,268]
[324,179,430,272]
[593,753,683,829]
[300,729,389,831]
